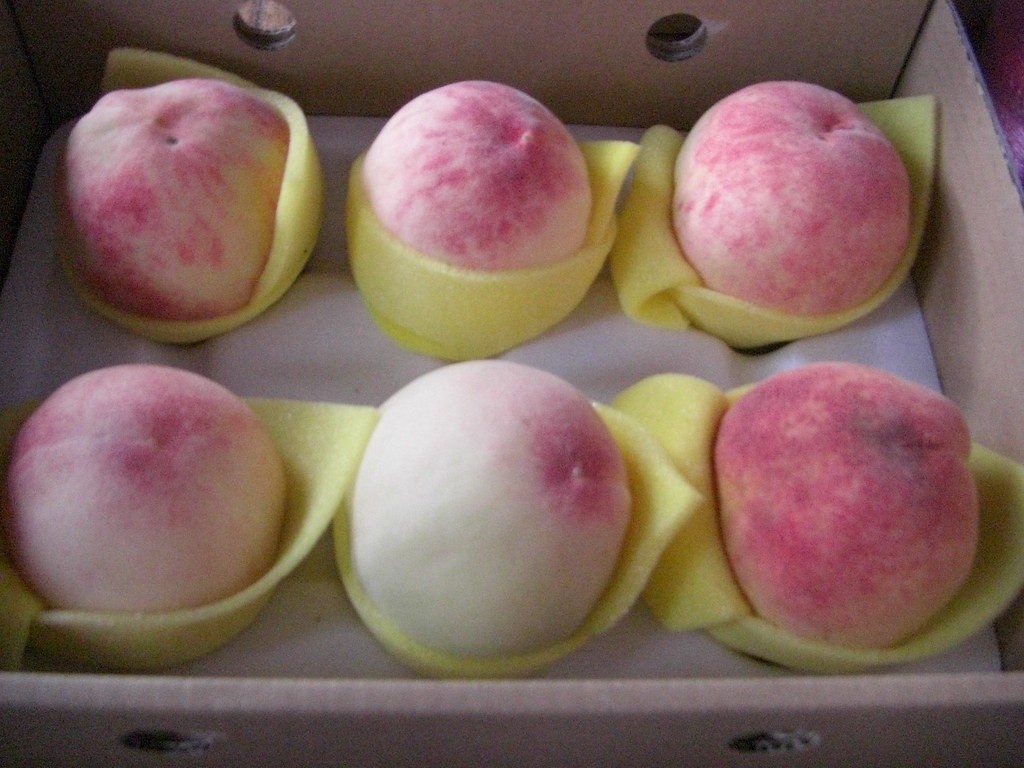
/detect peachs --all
[0,75,981,661]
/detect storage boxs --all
[5,0,1022,768]
